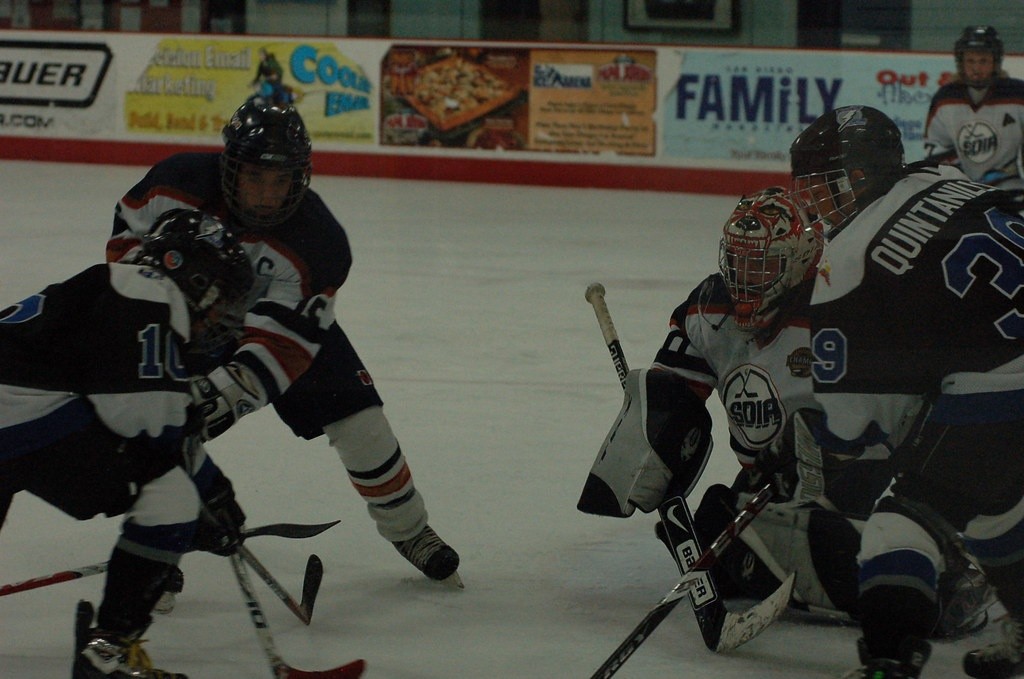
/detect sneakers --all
[71,601,187,679]
[392,524,465,589]
[962,616,1024,679]
[852,634,932,679]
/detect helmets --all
[717,185,823,331]
[218,97,309,226]
[135,208,253,321]
[954,25,1003,88]
[790,106,907,241]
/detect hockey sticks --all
[586,278,801,654]
[242,518,342,541]
[591,457,797,679]
[199,501,323,628]
[229,550,368,679]
[0,560,113,597]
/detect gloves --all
[192,361,260,445]
[191,452,246,527]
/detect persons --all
[578,26,1024,679]
[0,97,464,679]
